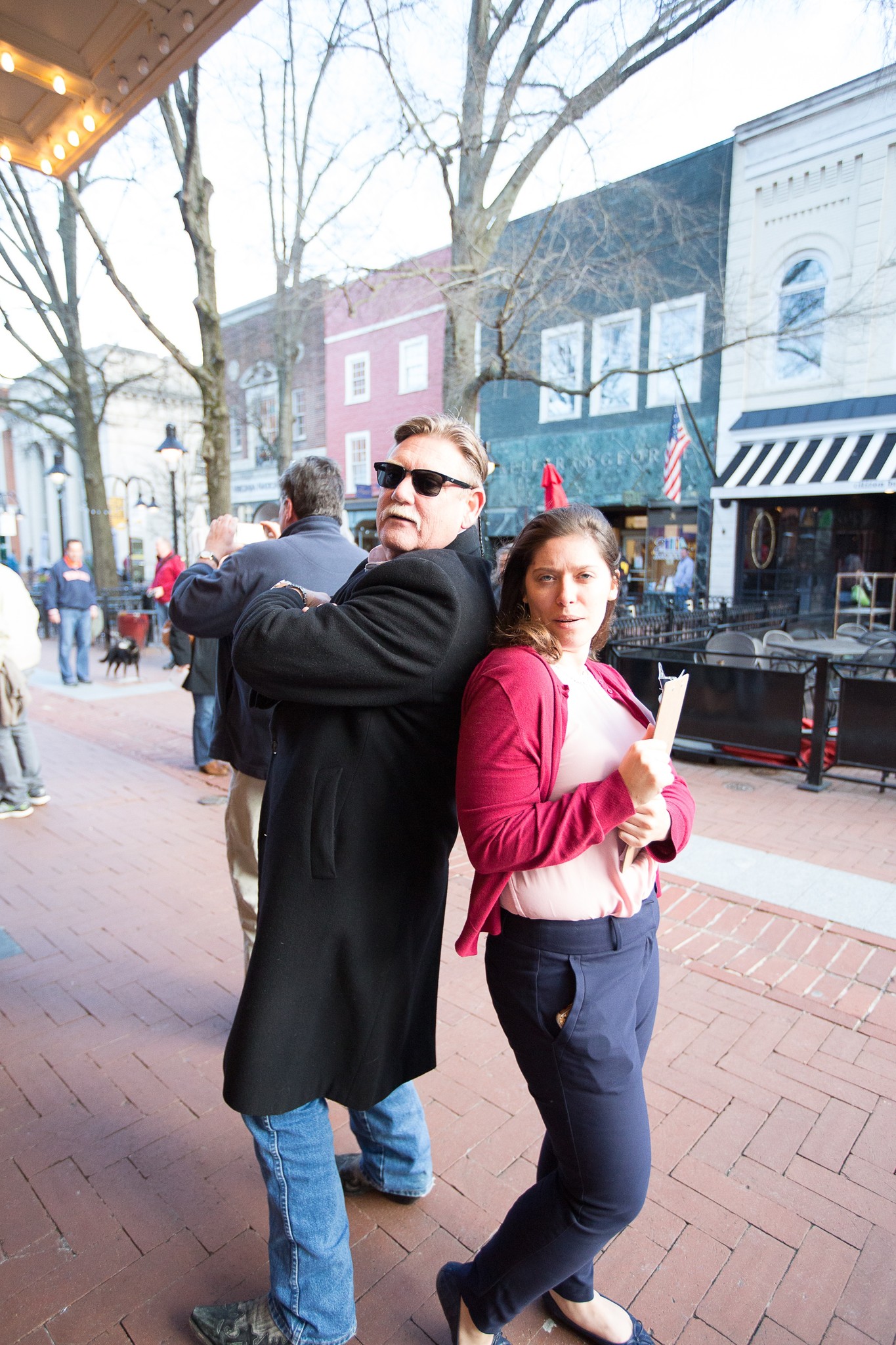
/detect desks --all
[766,638,896,793]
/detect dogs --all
[99,636,140,678]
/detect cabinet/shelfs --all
[834,572,896,639]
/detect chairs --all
[705,621,896,721]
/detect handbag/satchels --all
[162,620,195,655]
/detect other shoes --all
[333,1154,421,1205]
[29,786,51,805]
[163,660,176,670]
[63,677,78,685]
[189,1294,295,1345]
[0,800,34,819]
[435,1261,512,1345]
[200,760,230,775]
[77,674,92,684]
[543,1286,656,1345]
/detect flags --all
[661,397,691,504]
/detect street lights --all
[42,453,73,557]
[155,424,189,555]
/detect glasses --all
[374,462,474,496]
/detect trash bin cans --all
[117,614,149,647]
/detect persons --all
[42,540,99,685]
[831,554,872,623]
[146,537,188,671]
[0,562,51,821]
[672,537,694,612]
[490,543,515,610]
[170,622,232,777]
[185,415,498,1345]
[168,455,369,986]
[435,500,697,1345]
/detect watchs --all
[197,550,219,566]
[270,579,308,607]
